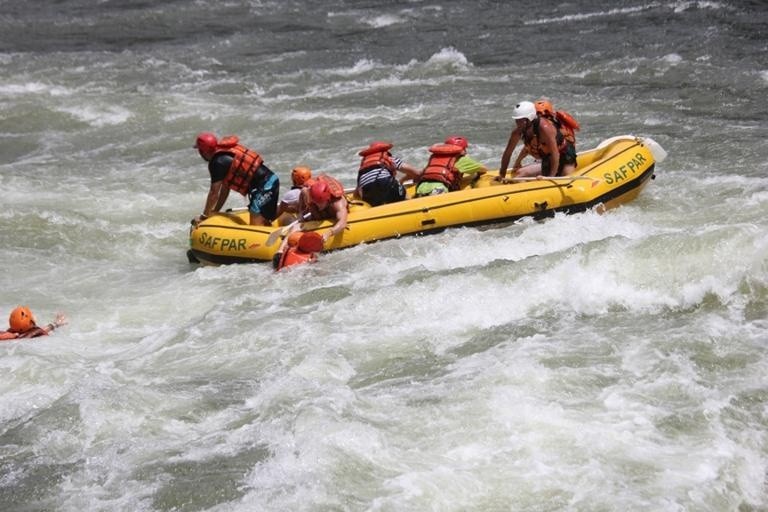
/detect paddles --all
[266,210,313,246]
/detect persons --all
[356,141,421,207]
[296,173,350,244]
[416,135,489,197]
[494,100,577,183]
[276,167,313,220]
[190,132,280,226]
[510,99,579,173]
[0,306,68,340]
[273,221,326,273]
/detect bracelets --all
[54,322,59,328]
[329,229,333,235]
[199,214,207,220]
[49,323,55,331]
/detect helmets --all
[9,306,33,332]
[287,231,304,247]
[310,182,331,203]
[535,100,553,113]
[192,133,218,151]
[511,101,537,121]
[292,167,311,185]
[445,137,468,148]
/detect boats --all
[188,134,656,265]
